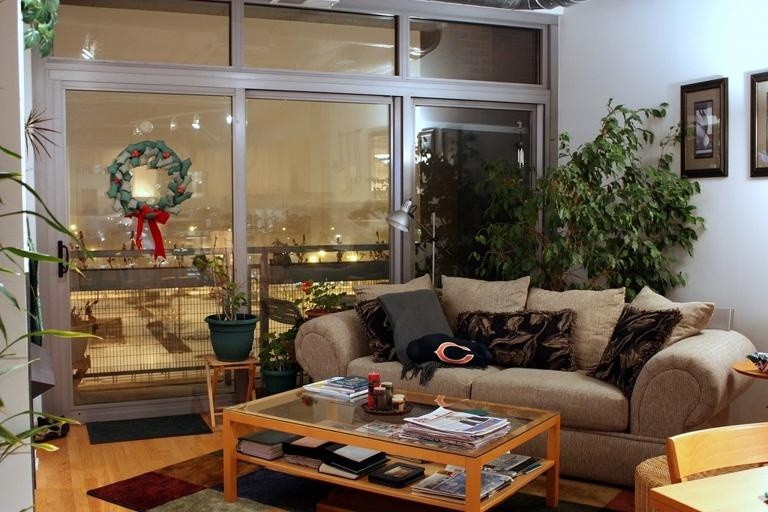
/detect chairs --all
[659,418,768,487]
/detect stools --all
[626,448,759,512]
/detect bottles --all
[367,373,393,410]
[391,393,406,412]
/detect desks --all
[643,464,768,511]
[726,352,768,386]
[198,350,262,430]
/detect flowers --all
[291,279,346,314]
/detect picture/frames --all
[749,69,768,179]
[676,74,732,179]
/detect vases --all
[305,308,342,321]
[69,321,96,364]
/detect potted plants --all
[196,234,264,364]
[254,327,301,395]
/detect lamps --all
[382,197,442,283]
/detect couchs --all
[288,296,763,494]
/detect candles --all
[367,370,407,413]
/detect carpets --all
[82,410,214,446]
[80,443,637,511]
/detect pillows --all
[377,284,459,368]
[355,295,401,363]
[520,280,629,371]
[348,272,438,307]
[450,302,580,376]
[584,303,684,398]
[438,270,534,330]
[623,283,718,349]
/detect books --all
[236,430,302,461]
[283,435,391,478]
[303,375,368,397]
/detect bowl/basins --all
[746,351,768,374]
